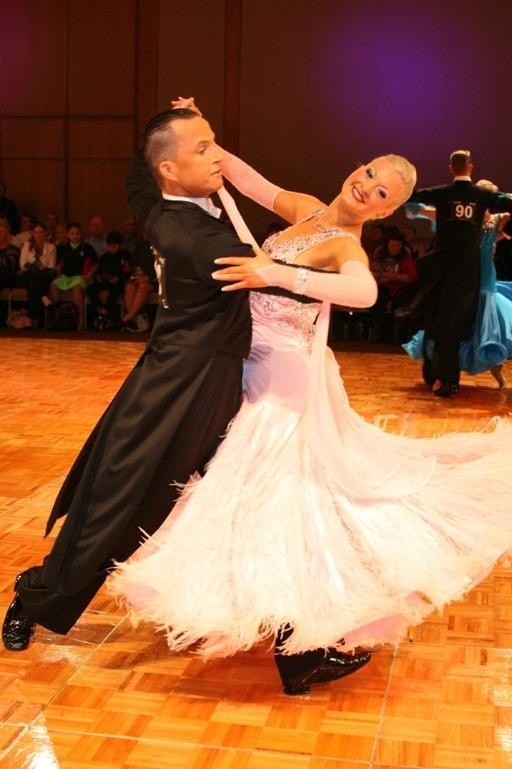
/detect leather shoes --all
[424,375,459,397]
[274,647,371,695]
[2,574,36,651]
